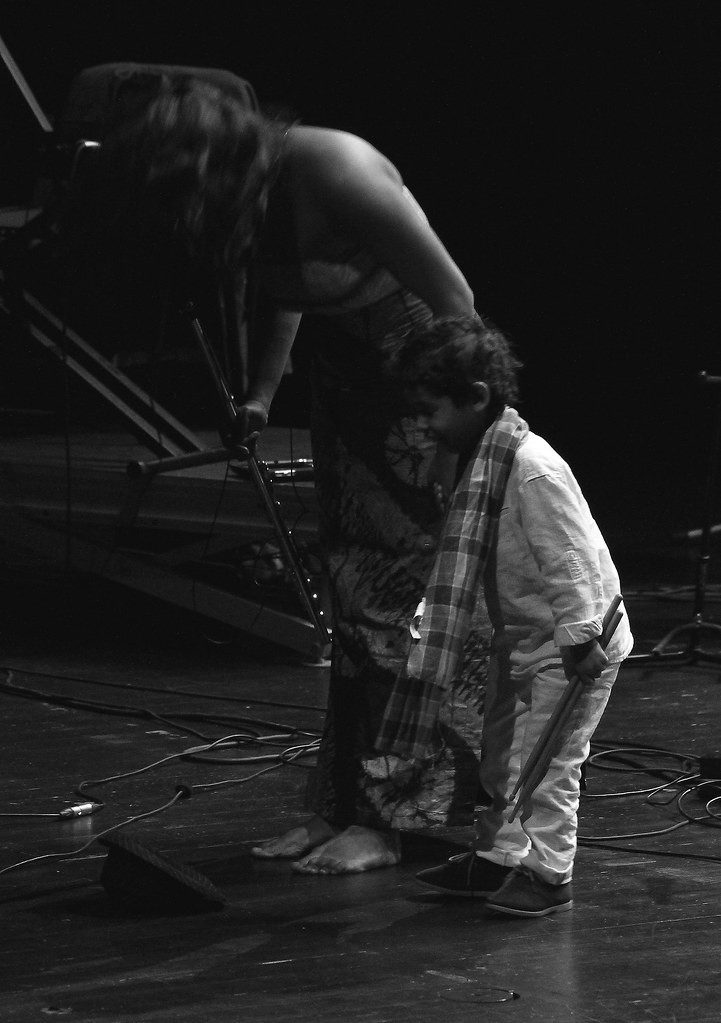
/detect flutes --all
[181,296,332,646]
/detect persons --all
[395,312,638,920]
[100,57,511,880]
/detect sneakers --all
[488,867,575,917]
[413,853,512,898]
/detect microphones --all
[126,444,251,477]
